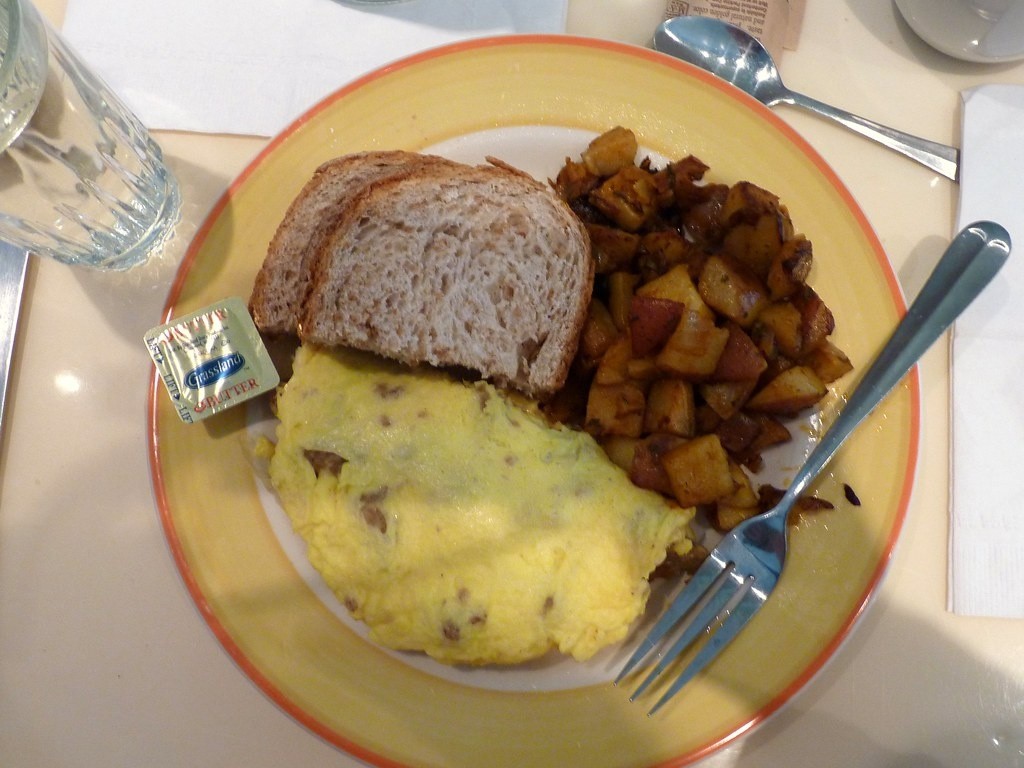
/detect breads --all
[246,148,475,338]
[298,171,596,391]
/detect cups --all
[0,0,182,272]
[895,0,1024,62]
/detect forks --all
[613,221,1012,717]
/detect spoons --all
[653,16,961,185]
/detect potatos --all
[538,126,856,538]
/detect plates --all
[149,36,921,768]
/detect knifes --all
[0,241,29,424]
